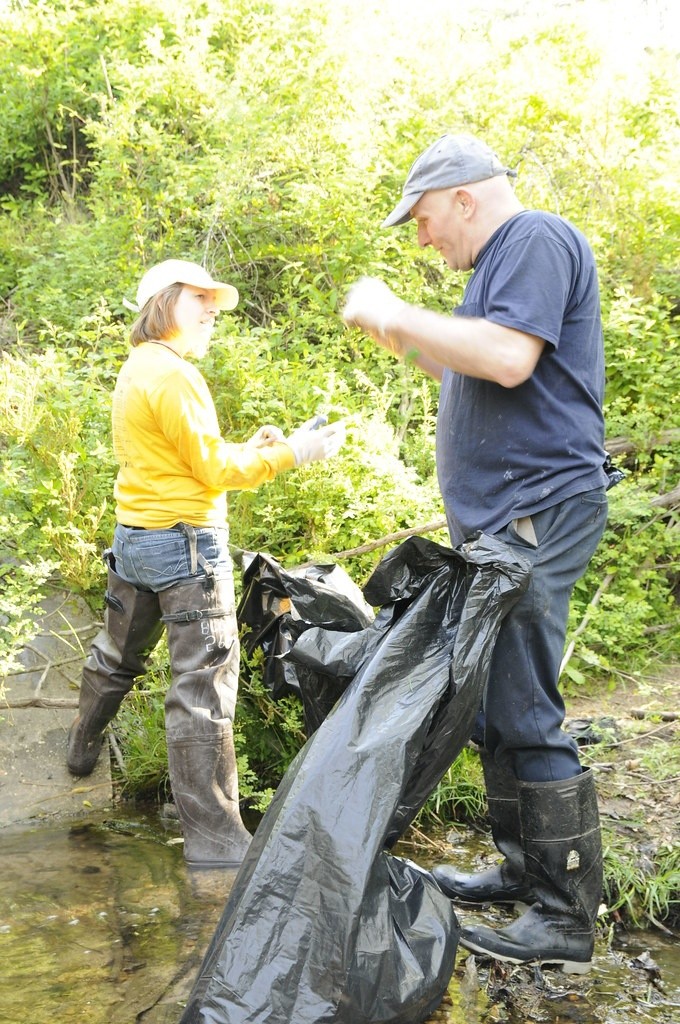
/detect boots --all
[459,766,602,974]
[68,549,167,775]
[158,554,253,866]
[432,786,538,914]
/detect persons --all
[66,257,340,867]
[341,132,608,976]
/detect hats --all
[123,260,239,313]
[379,133,517,228]
